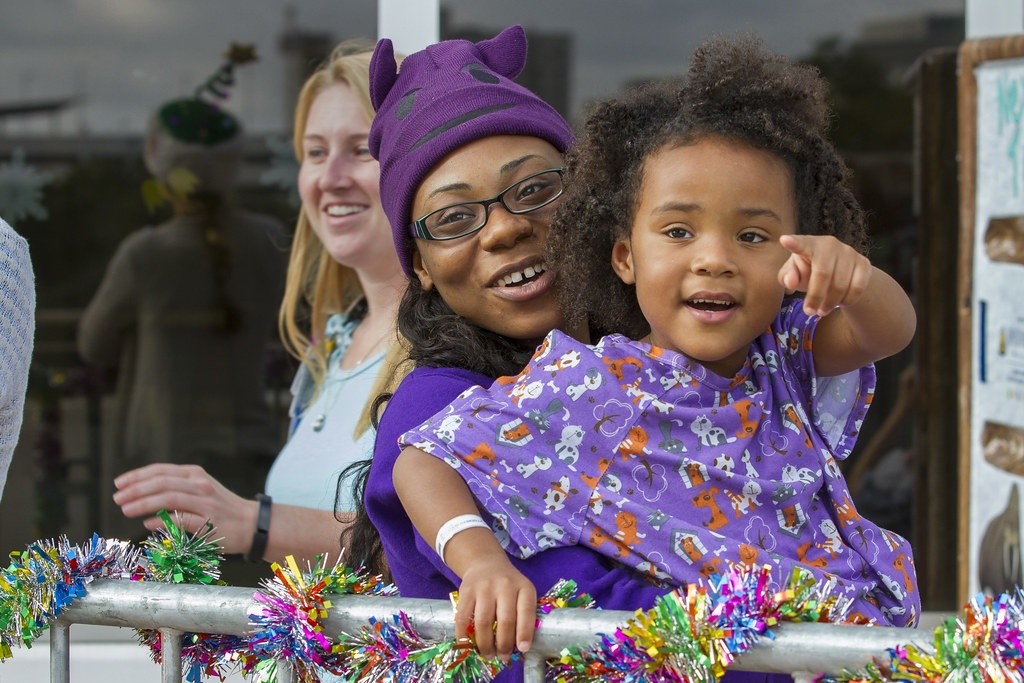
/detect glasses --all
[407,167,576,246]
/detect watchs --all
[247,490,275,565]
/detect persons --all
[390,28,921,665]
[335,70,802,682]
[113,41,411,595]
[74,97,291,477]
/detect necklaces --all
[313,302,389,434]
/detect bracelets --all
[435,514,492,563]
